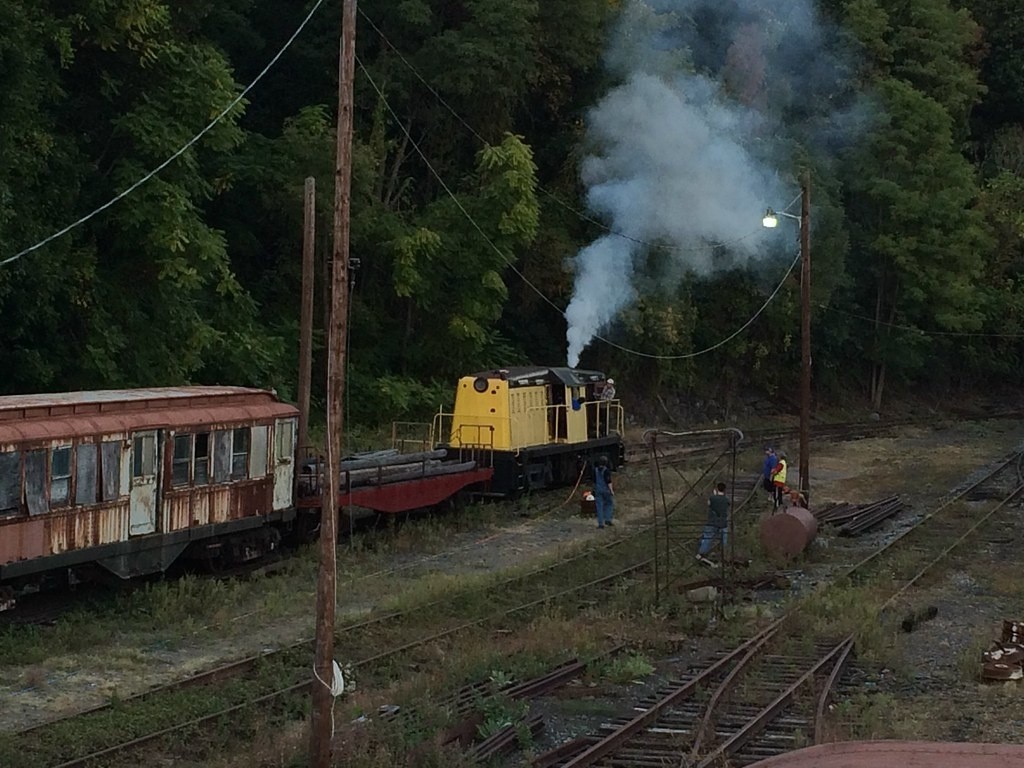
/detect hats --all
[606,378,615,385]
[776,450,788,458]
[599,455,608,461]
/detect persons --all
[696,483,731,558]
[763,446,787,515]
[572,397,586,411]
[598,378,616,437]
[593,456,615,529]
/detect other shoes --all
[598,525,604,529]
[605,520,613,526]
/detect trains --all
[0,364,629,593]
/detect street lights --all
[760,168,813,511]
